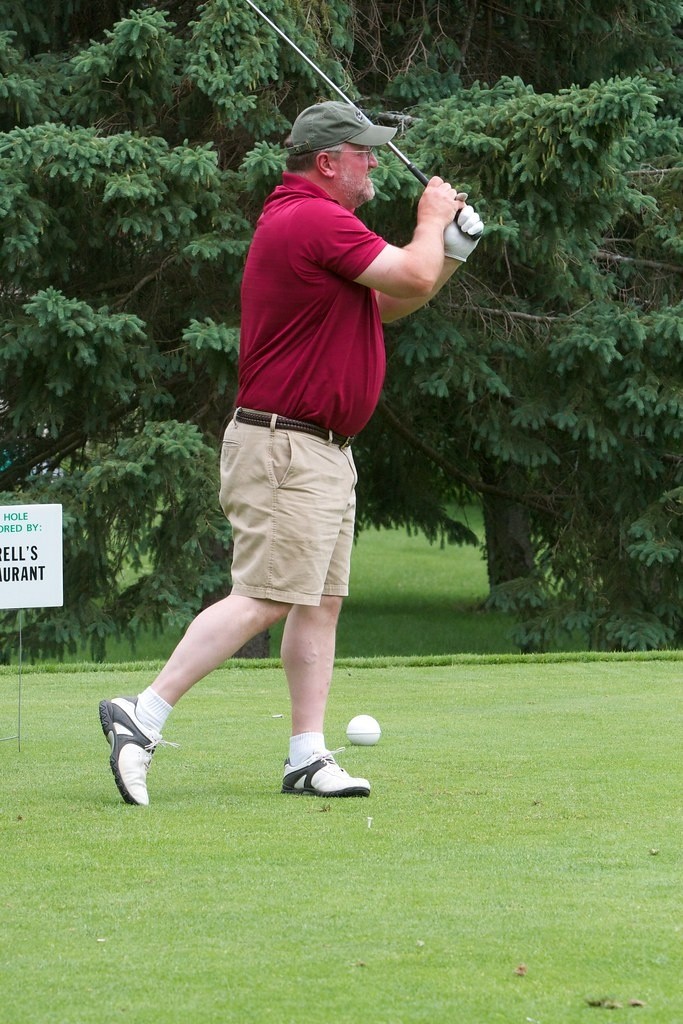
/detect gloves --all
[443,193,484,263]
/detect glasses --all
[330,147,374,160]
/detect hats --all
[288,100,398,155]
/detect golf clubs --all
[242,0,484,241]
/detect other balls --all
[345,714,382,747]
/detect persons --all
[99,98,482,806]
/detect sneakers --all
[99,695,180,805]
[281,747,370,797]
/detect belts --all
[236,411,355,449]
